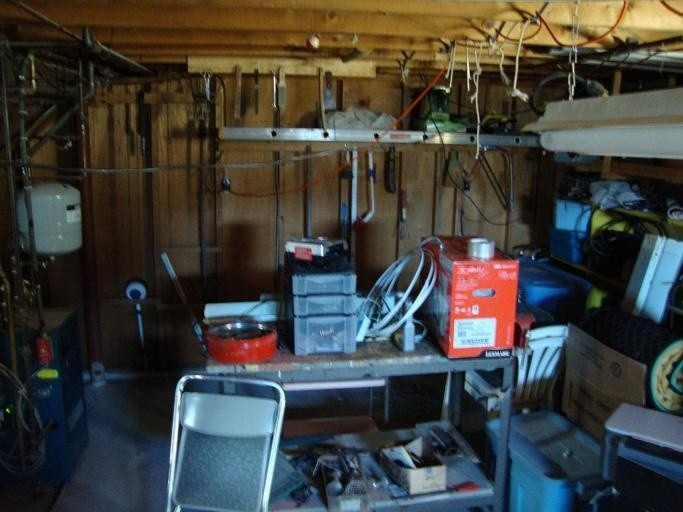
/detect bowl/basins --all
[204,321,277,365]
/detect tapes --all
[467,237,496,259]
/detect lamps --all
[521,86,682,161]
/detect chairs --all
[166,374,286,512]
[484,325,568,415]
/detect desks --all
[205,340,516,512]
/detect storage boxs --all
[562,323,648,443]
[477,410,601,512]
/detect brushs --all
[442,149,464,188]
